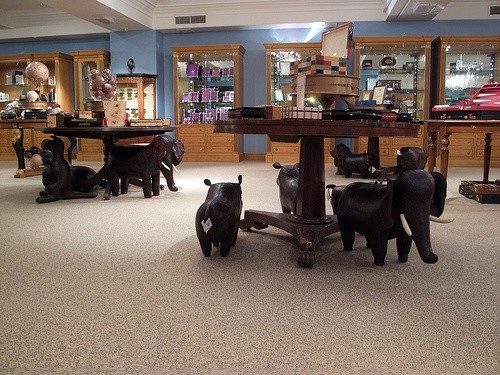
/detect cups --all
[102,100,127,128]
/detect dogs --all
[330,143,370,179]
[34,137,98,204]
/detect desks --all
[42,126,175,200]
[0,118,98,170]
[211,120,420,267]
[427,119,500,182]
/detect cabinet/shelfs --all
[115,73,156,126]
[69,50,110,160]
[172,44,247,163]
[348,36,433,168]
[262,43,352,163]
[0,52,75,162]
[432,36,500,168]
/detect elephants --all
[102,134,186,199]
[327,169,456,267]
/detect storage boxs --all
[291,22,354,76]
[263,106,282,119]
[459,180,500,204]
